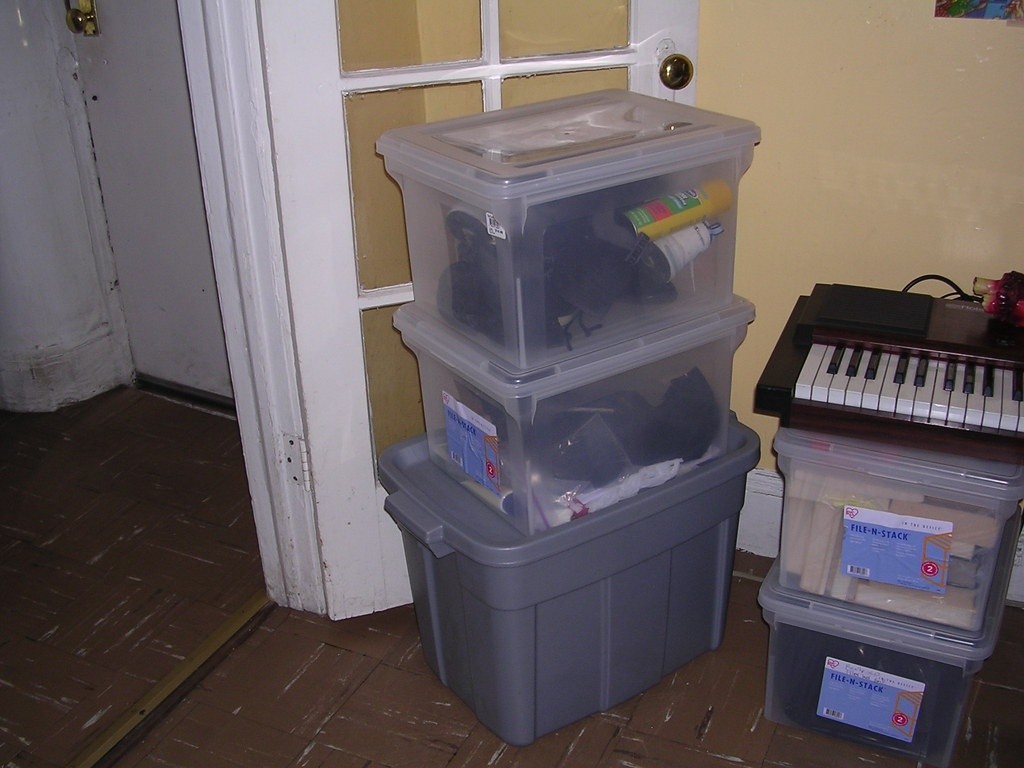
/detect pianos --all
[752,283,1024,465]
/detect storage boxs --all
[375,89,763,747]
[758,427,1024,768]
